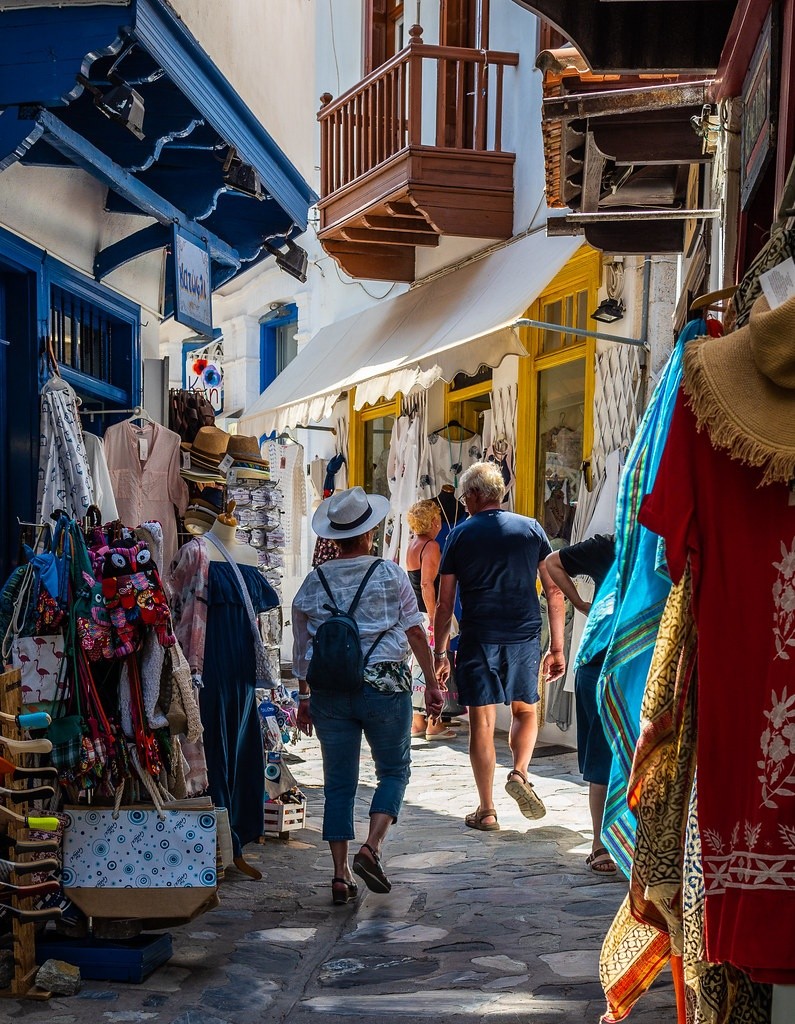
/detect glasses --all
[458,487,478,507]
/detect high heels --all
[331,878,361,902]
[353,843,393,893]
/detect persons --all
[432,462,567,831]
[429,484,470,651]
[289,487,445,907]
[163,514,281,855]
[545,529,620,877]
[405,501,457,741]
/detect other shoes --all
[442,720,462,726]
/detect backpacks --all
[307,558,397,690]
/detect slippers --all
[426,729,458,740]
[411,728,426,737]
[168,388,216,441]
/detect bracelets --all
[298,693,310,699]
[433,649,446,659]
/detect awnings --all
[239,226,647,440]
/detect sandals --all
[505,770,546,821]
[585,847,617,875]
[465,804,500,831]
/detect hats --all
[680,293,795,484]
[312,486,391,539]
[176,426,271,538]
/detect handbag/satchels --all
[436,649,468,717]
[449,614,459,640]
[1,514,233,985]
[253,639,283,689]
[726,225,795,331]
